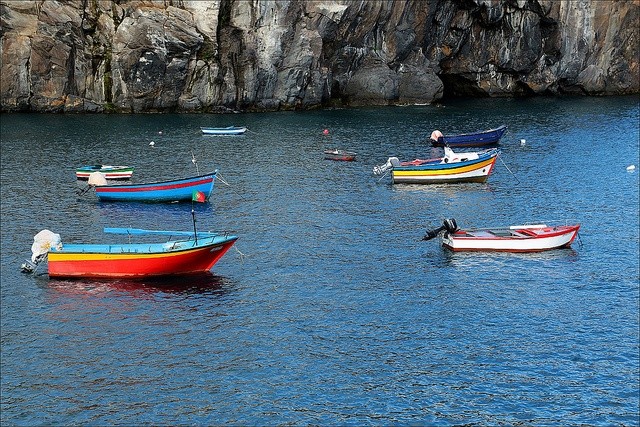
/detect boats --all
[431,124,508,145]
[200,124,247,136]
[425,218,580,252]
[47,235,239,279]
[323,147,356,160]
[96,168,217,202]
[388,147,500,184]
[76,162,134,179]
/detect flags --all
[192,189,205,202]
